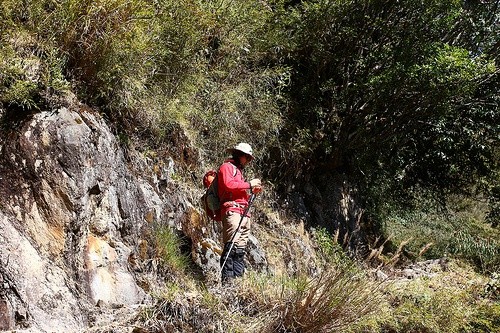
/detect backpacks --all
[201,174,222,221]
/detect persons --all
[205,142,261,290]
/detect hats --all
[225,143,256,160]
